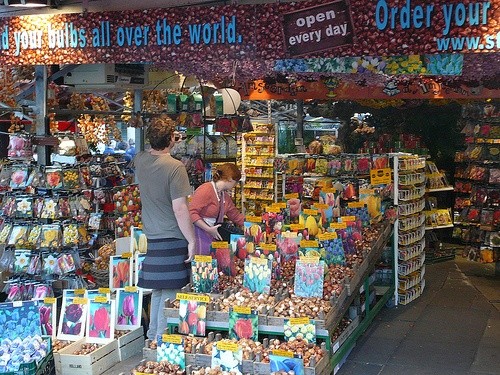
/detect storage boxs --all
[53,326,330,375]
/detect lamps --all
[214,88,241,114]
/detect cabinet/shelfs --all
[425,187,455,264]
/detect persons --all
[132,119,197,349]
[188,162,249,260]
